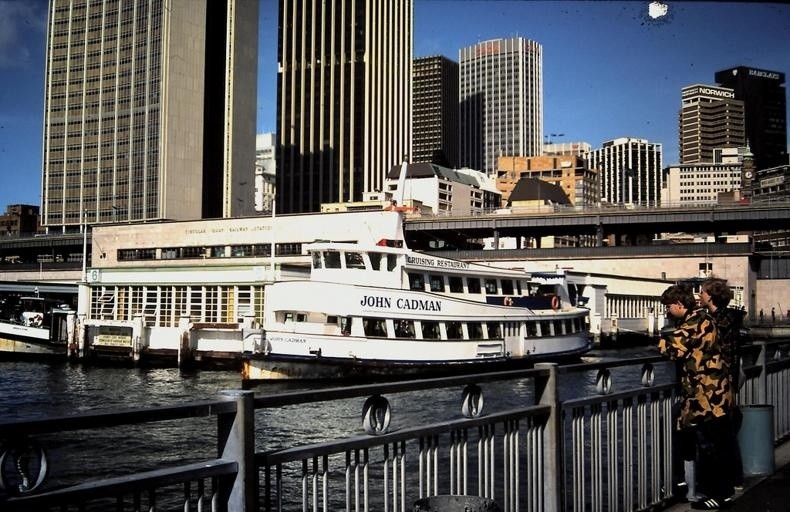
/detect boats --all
[241,242,595,379]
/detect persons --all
[656,283,743,510]
[698,278,748,489]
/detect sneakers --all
[691,483,744,510]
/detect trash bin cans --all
[737,404,776,478]
[412,495,500,512]
